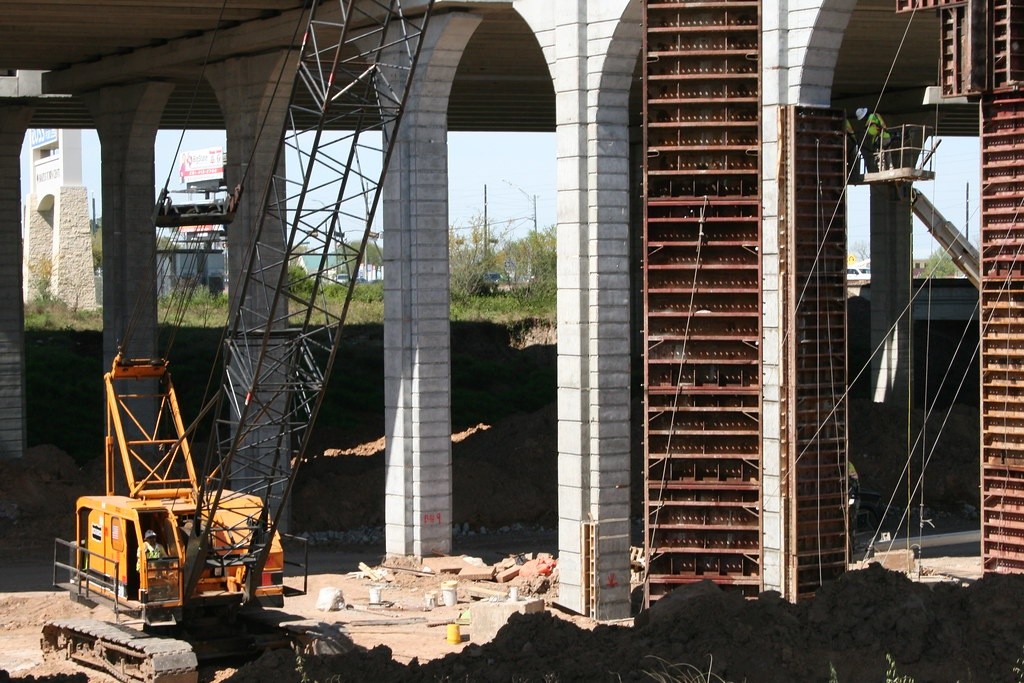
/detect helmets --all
[856,108,867,120]
[145,530,156,539]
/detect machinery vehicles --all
[37,1,436,682]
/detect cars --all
[465,273,510,297]
[336,273,349,284]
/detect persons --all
[847,120,857,169]
[135,530,174,600]
[848,462,860,562]
[856,107,894,172]
